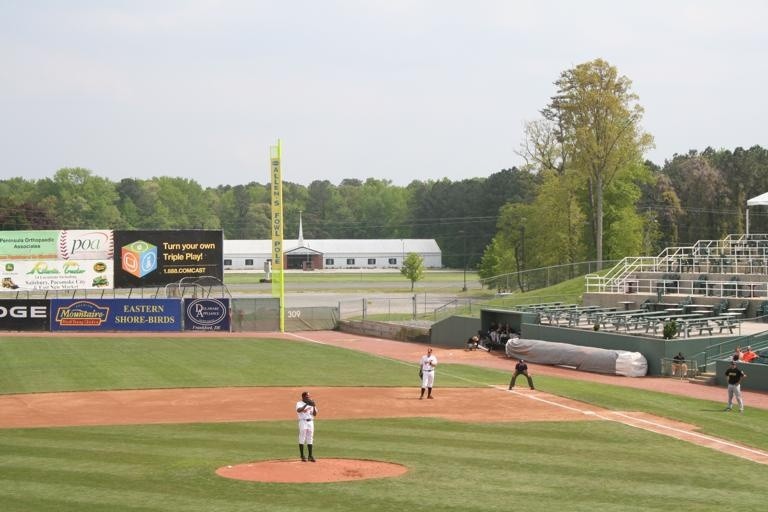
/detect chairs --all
[657,237,768,296]
[751,350,768,365]
[640,296,768,321]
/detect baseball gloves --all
[419,370,423,378]
[305,398,316,406]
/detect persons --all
[487,320,514,335]
[724,361,747,411]
[731,346,743,362]
[742,346,759,362]
[295,392,318,461]
[464,336,479,351]
[671,352,688,376]
[265,263,271,279]
[655,318,664,334]
[509,358,534,390]
[418,347,437,400]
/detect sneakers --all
[302,456,315,462]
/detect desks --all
[514,301,746,337]
